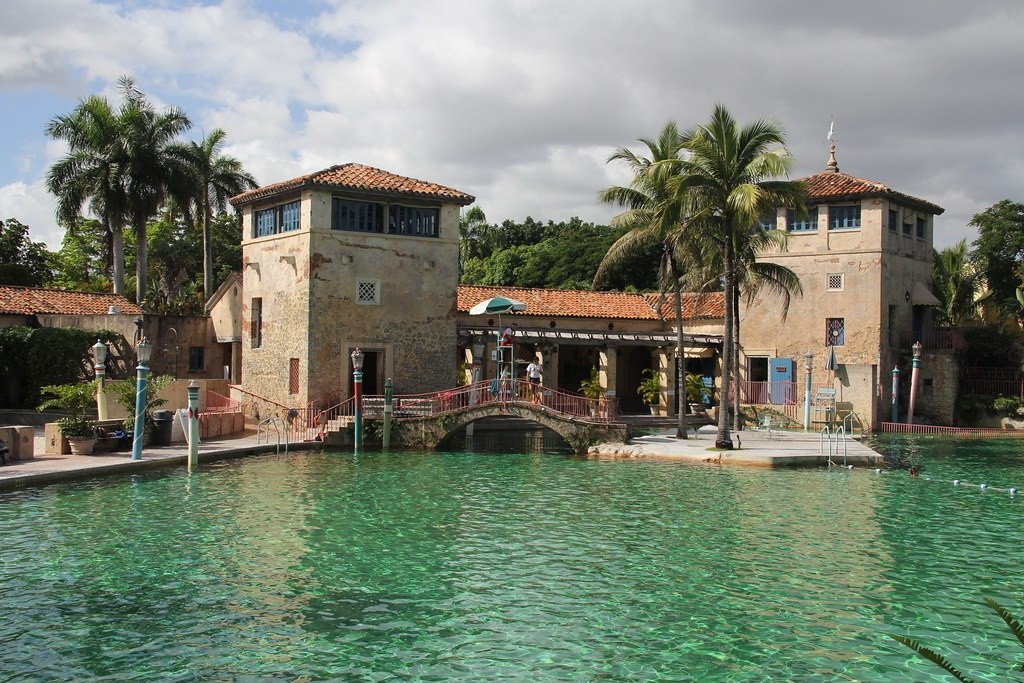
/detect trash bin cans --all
[152,409,173,447]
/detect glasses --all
[534,359,537,360]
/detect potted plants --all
[107,372,175,447]
[636,368,661,416]
[34,379,103,455]
[580,366,605,417]
[685,371,713,414]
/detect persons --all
[501,366,512,397]
[527,356,543,405]
[502,323,518,347]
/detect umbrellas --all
[469,297,526,398]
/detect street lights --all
[132,334,153,459]
[906,339,922,424]
[351,347,365,454]
[803,349,814,431]
[92,340,108,420]
